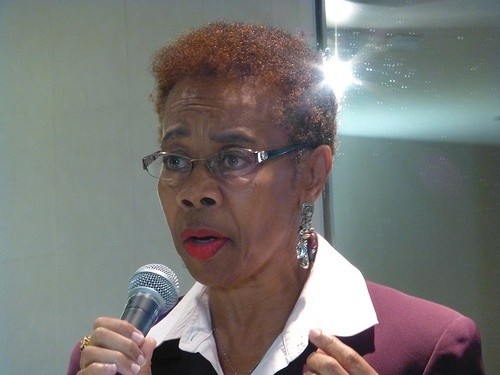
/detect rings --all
[80,335,91,351]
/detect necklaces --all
[211,325,259,375]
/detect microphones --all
[118,264,180,336]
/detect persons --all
[65,20,485,375]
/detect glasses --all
[141,144,309,181]
